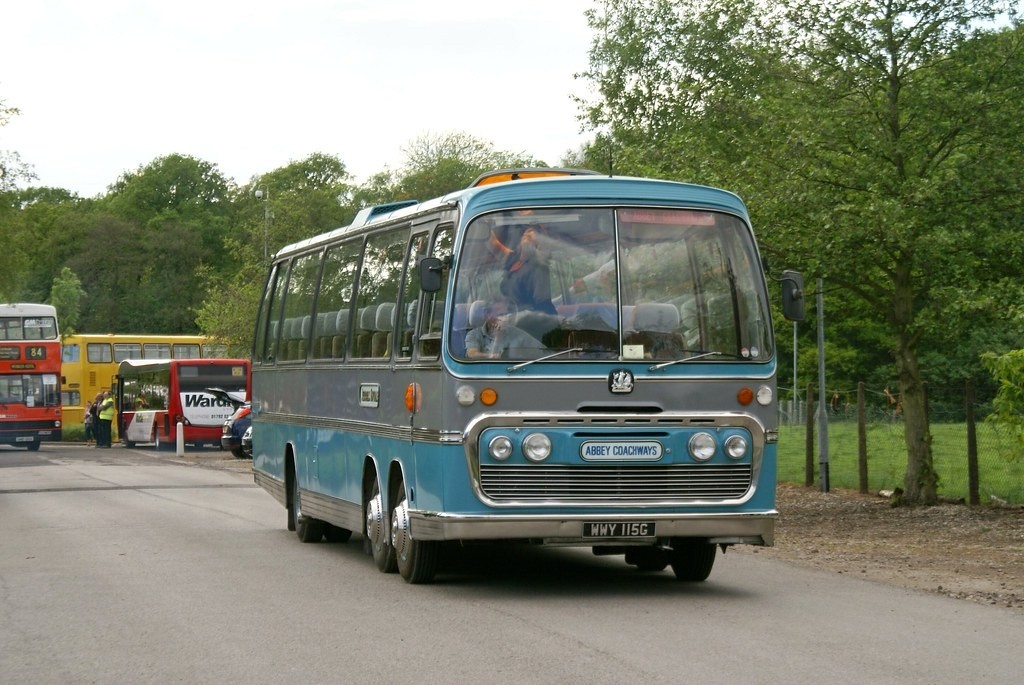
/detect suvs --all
[204,388,253,459]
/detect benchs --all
[265,302,691,361]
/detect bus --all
[111,358,251,452]
[47,333,246,428]
[243,165,806,591]
[0,303,67,452]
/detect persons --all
[83,400,96,447]
[97,391,114,448]
[89,393,102,447]
[464,297,556,358]
[134,391,145,410]
[484,209,558,315]
[140,389,149,409]
